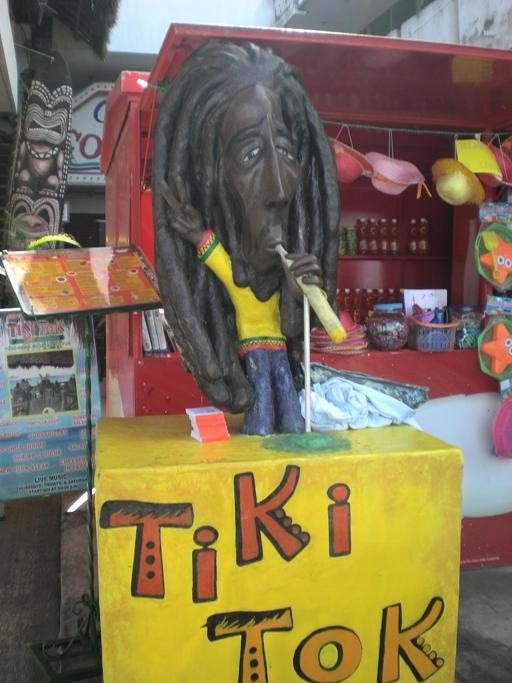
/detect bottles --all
[334,216,429,256]
[335,287,405,320]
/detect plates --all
[309,320,369,357]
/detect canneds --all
[338,227,357,256]
